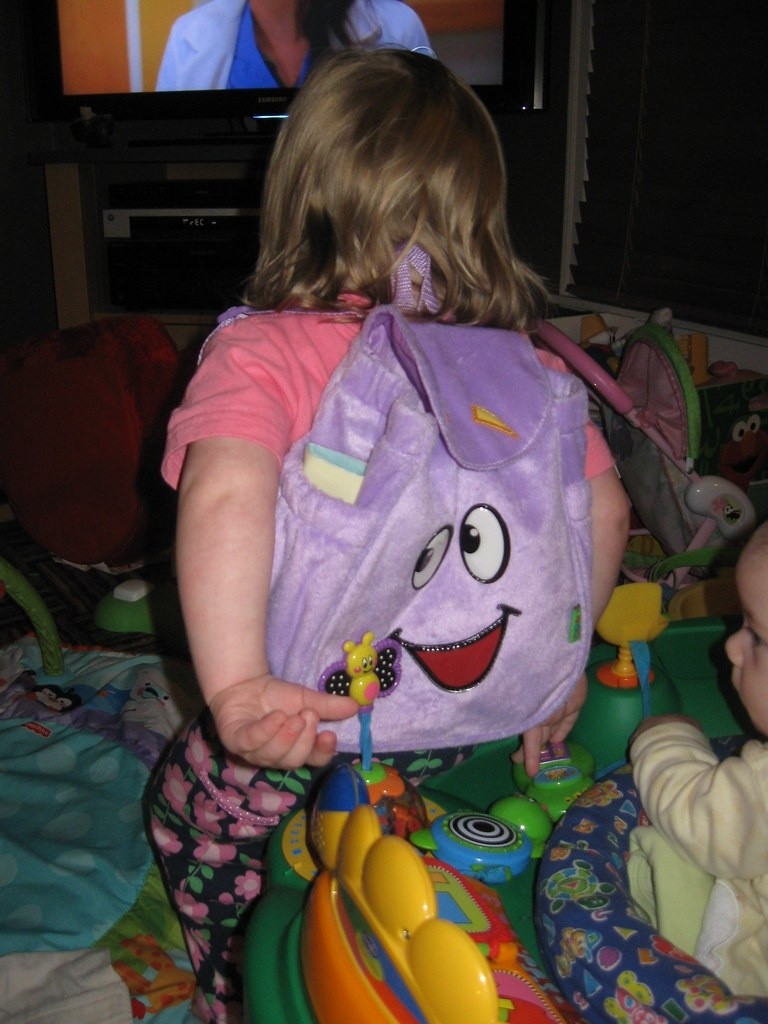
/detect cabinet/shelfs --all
[44,163,259,342]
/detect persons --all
[148,45,632,1024]
[628,520,768,998]
[152,0,431,92]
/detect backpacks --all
[265,242,596,753]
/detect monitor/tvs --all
[16,1,544,138]
